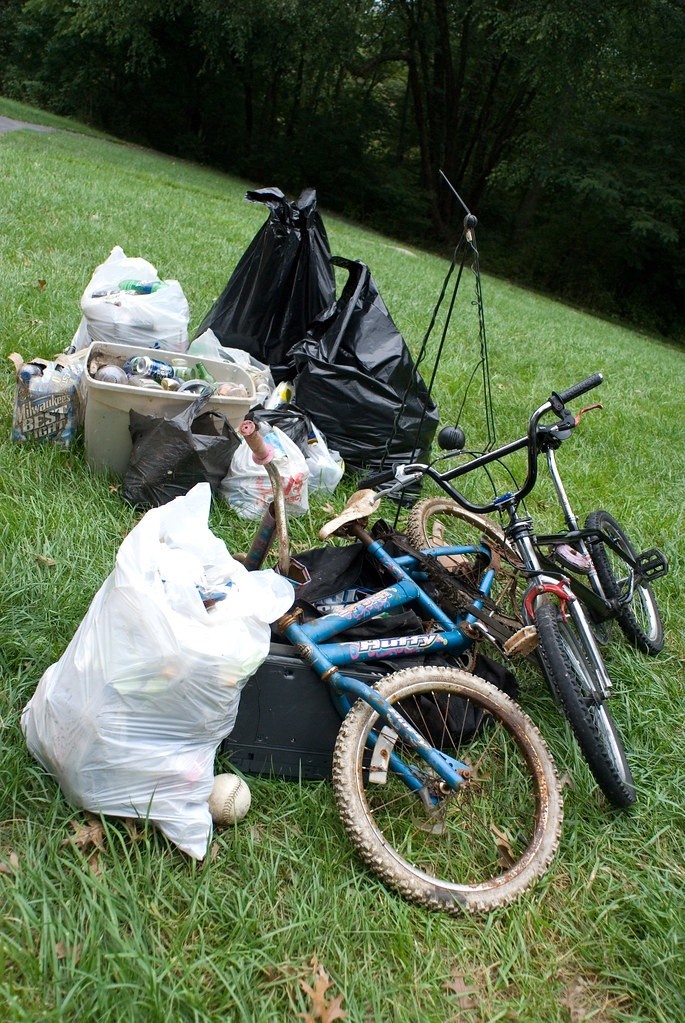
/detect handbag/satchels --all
[214,543,521,781]
[217,402,344,522]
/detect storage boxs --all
[220,653,421,783]
[83,340,256,477]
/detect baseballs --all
[206,773,252,825]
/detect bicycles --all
[356,371,668,809]
[231,420,565,918]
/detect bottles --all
[197,361,216,383]
[118,279,167,294]
[66,346,76,355]
[90,288,139,299]
[95,364,128,385]
[130,375,164,390]
[19,364,42,380]
